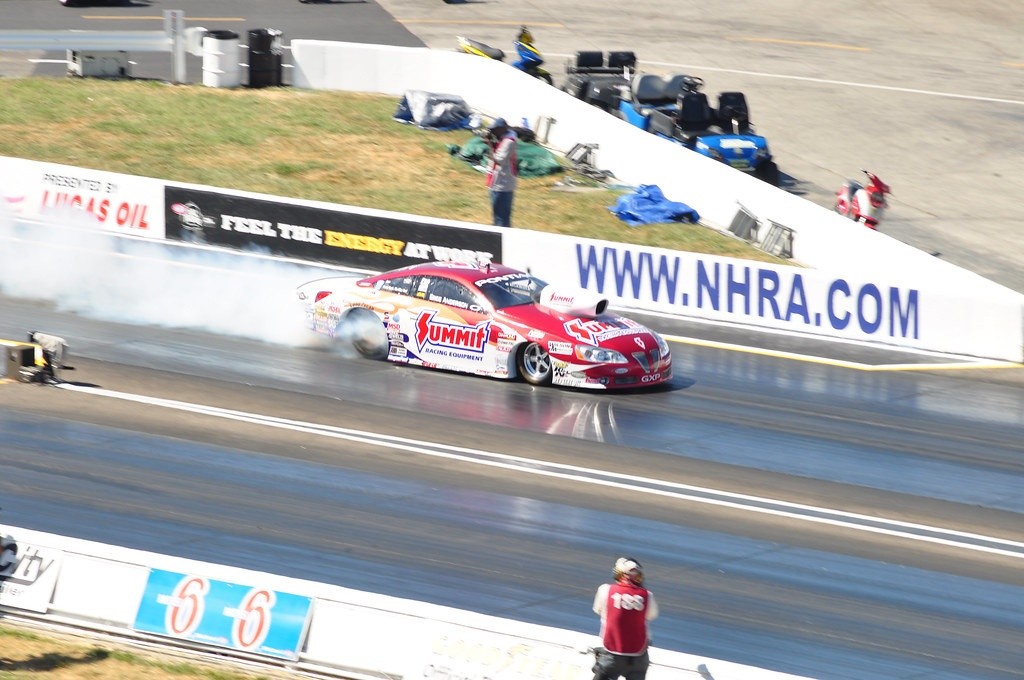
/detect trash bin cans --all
[203,30,242,88]
[248,27,283,87]
[0,340,35,379]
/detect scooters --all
[456,25,553,86]
[835,169,892,232]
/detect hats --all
[487,117,507,129]
[615,558,642,574]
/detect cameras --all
[485,131,494,140]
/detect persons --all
[481,117,516,227]
[591,556,658,680]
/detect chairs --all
[670,94,718,141]
[574,51,700,115]
[719,90,748,132]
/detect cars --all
[296,261,672,392]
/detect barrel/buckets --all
[202,30,240,88]
[247,29,280,87]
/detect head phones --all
[613,558,641,585]
